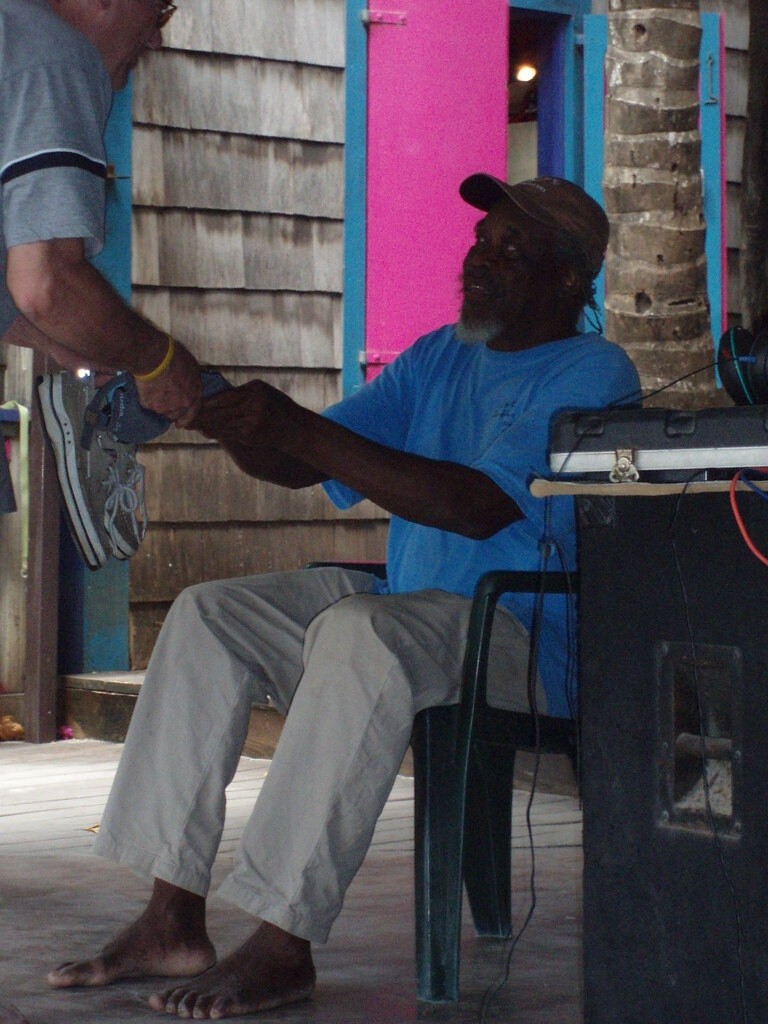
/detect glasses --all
[155,0,177,28]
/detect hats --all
[459,173,609,281]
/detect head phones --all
[718,325,768,405]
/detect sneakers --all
[35,369,138,571]
[97,429,149,562]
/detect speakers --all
[572,474,768,1024]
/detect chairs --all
[304,560,578,1006]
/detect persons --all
[1,0,201,432]
[48,171,643,1019]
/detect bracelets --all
[133,335,174,382]
